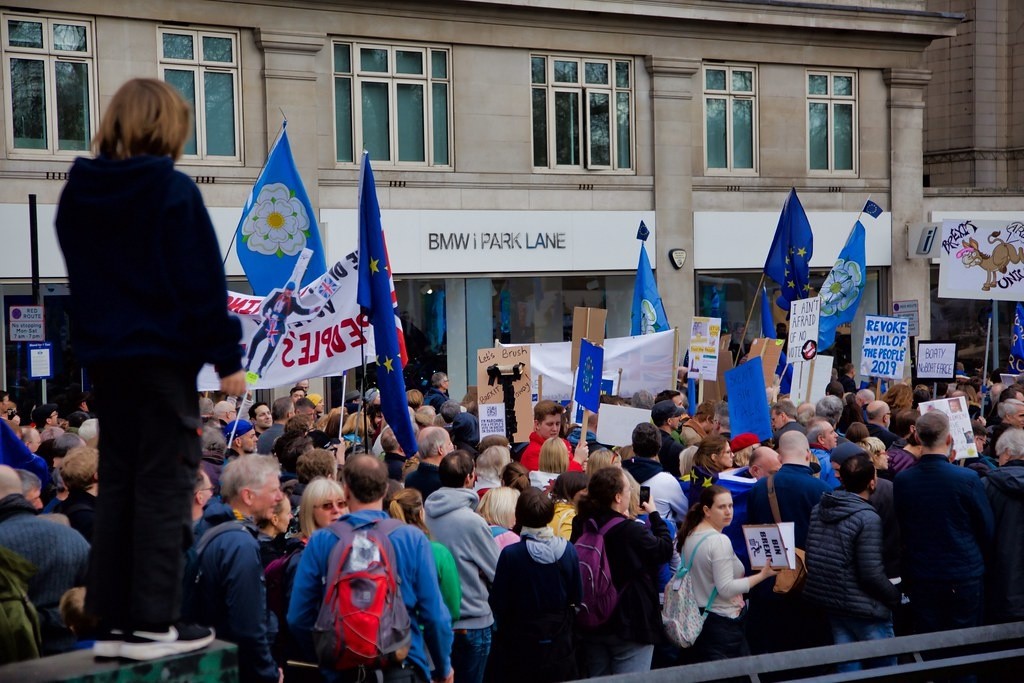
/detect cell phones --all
[639,486,650,509]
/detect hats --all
[306,430,340,451]
[305,393,323,406]
[223,419,255,445]
[730,433,761,453]
[364,387,380,402]
[651,400,685,425]
[344,389,360,403]
[32,403,59,423]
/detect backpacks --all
[660,528,718,650]
[311,518,412,683]
[264,548,303,629]
[573,517,625,631]
[176,521,253,624]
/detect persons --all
[244,281,321,378]
[54,78,247,661]
[0,391,99,665]
[950,399,961,413]
[728,322,752,366]
[963,428,974,443]
[692,322,705,336]
[185,361,1024,683]
[776,323,788,354]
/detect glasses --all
[875,450,889,457]
[49,412,59,418]
[228,410,237,415]
[305,423,317,432]
[977,437,989,448]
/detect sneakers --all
[93,622,126,662]
[118,619,216,662]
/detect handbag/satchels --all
[773,548,808,595]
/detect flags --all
[862,199,883,219]
[355,150,418,458]
[811,221,865,351]
[636,220,649,241]
[762,188,813,302]
[1008,303,1024,374]
[711,282,729,331]
[760,284,794,396]
[265,320,280,347]
[630,246,670,336]
[236,120,328,297]
[317,275,337,298]
